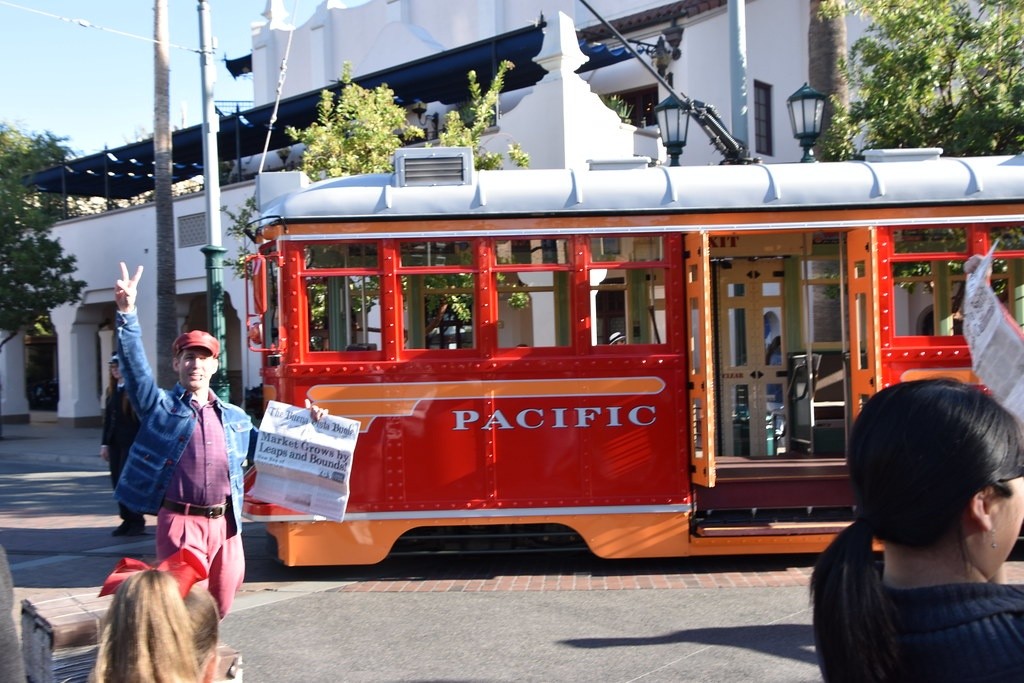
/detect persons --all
[964,255,1024,343]
[0,546,27,683]
[100,351,146,536]
[115,262,329,621]
[90,548,221,683]
[609,332,626,345]
[809,379,1024,683]
[766,336,783,402]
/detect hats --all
[172,330,221,359]
[609,332,625,345]
[108,351,119,364]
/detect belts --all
[162,499,231,519]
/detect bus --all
[237,0,1024,569]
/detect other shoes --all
[111,521,144,536]
